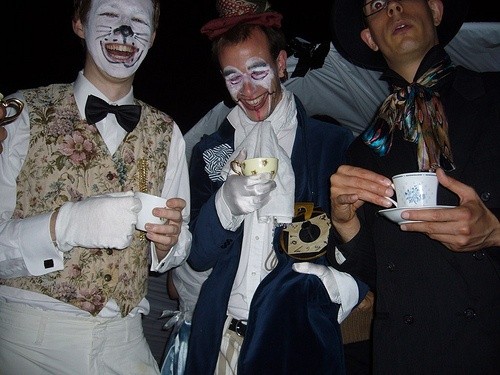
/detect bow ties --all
[84,94,141,132]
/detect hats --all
[197,0,285,35]
[329,0,466,70]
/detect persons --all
[329,0,500,375]
[189,1,377,375]
[0,0,193,375]
[161,261,213,375]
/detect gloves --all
[55,190,142,254]
[291,262,357,324]
[220,148,277,215]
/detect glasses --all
[361,1,389,18]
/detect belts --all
[226,315,247,337]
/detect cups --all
[133,192,173,232]
[230,158,278,182]
[384,173,439,207]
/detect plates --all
[378,205,457,225]
